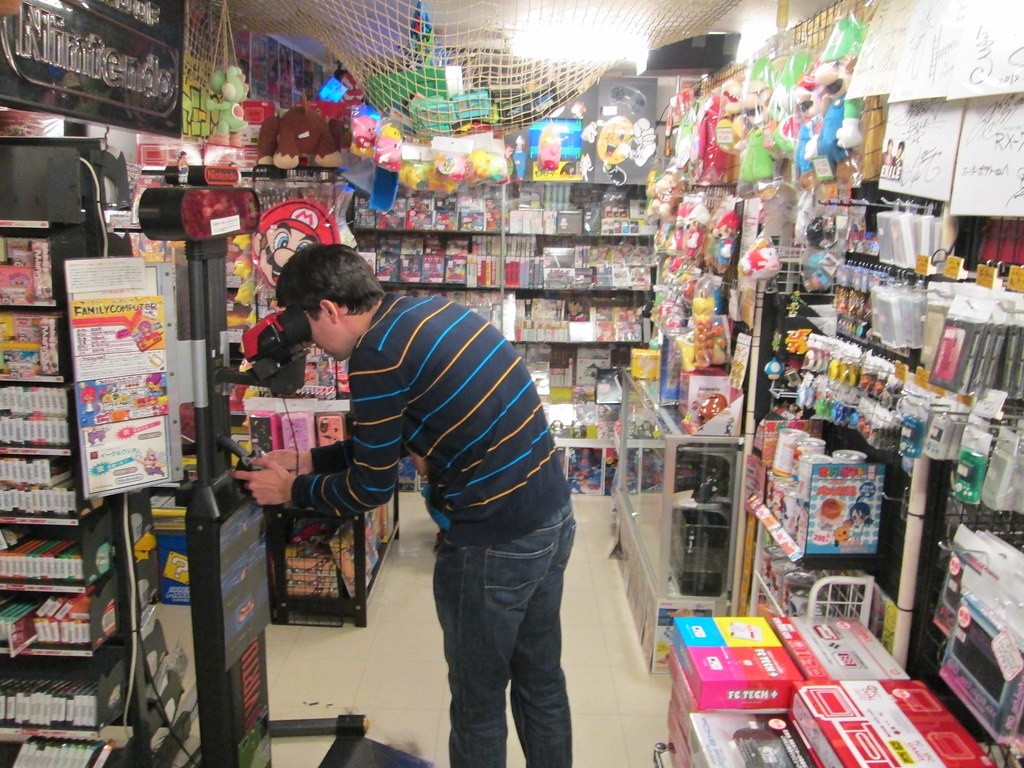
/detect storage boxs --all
[770,614,911,681]
[504,31,744,436]
[666,650,697,768]
[686,711,819,768]
[367,64,490,136]
[672,614,803,712]
[670,508,731,597]
[787,680,998,768]
[0,235,59,375]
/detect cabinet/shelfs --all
[113,161,401,628]
[350,180,657,347]
[0,135,191,768]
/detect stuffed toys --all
[646,163,781,279]
[732,61,861,182]
[256,105,352,169]
[207,66,249,147]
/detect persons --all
[880,138,906,180]
[178,151,188,187]
[229,243,576,768]
